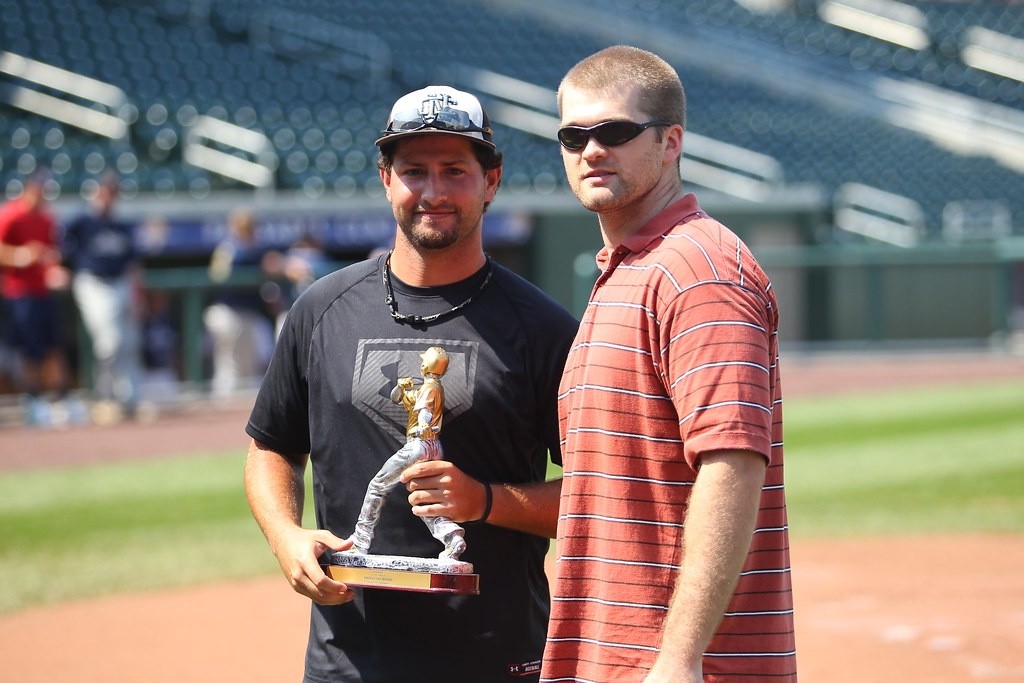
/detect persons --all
[242,84,581,682]
[60,171,156,427]
[1,171,77,428]
[198,197,336,405]
[533,44,797,683]
[341,346,467,561]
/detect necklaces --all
[381,246,495,328]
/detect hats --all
[374,84,496,152]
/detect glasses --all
[557,119,672,152]
[384,106,493,138]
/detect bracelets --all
[476,478,493,526]
